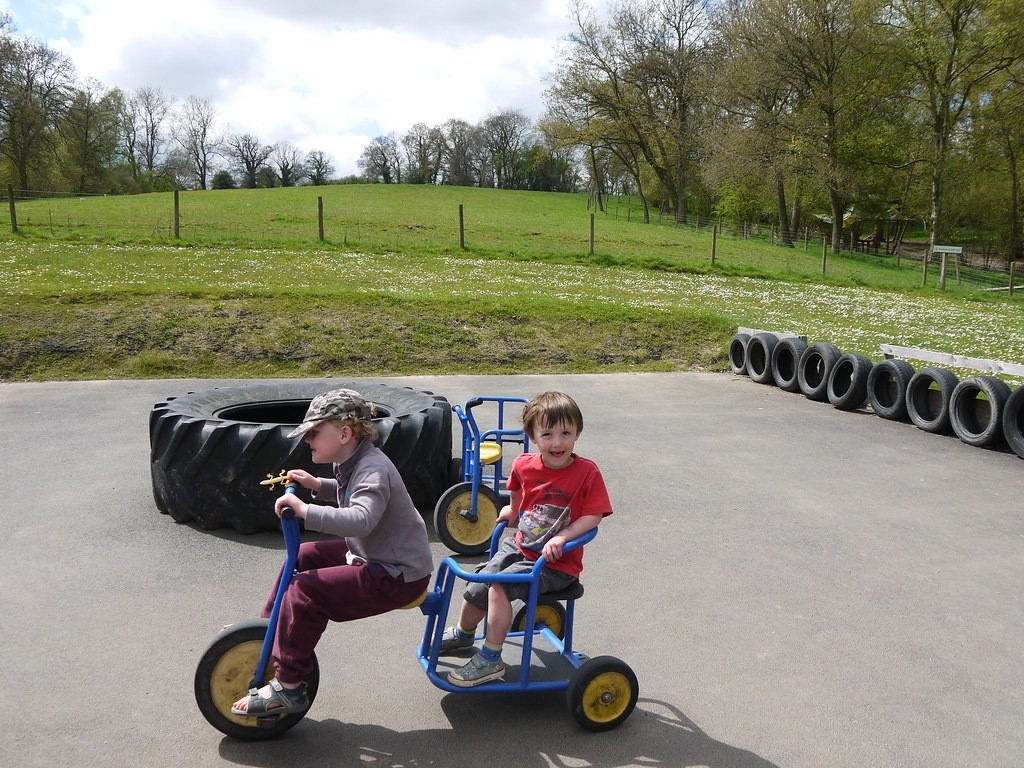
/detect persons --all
[230,387,434,717]
[423,390,614,687]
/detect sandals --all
[231,677,308,716]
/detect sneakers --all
[430,627,475,651]
[446,656,505,687]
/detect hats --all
[287,388,371,441]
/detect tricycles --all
[194,472,640,744]
[431,397,535,556]
